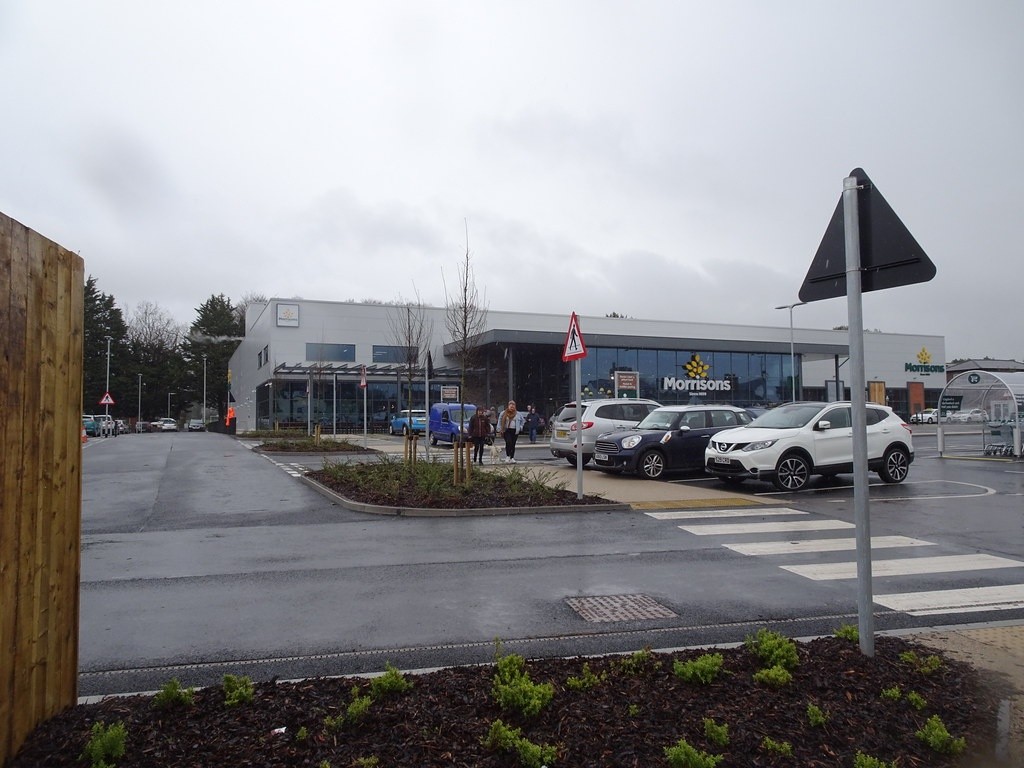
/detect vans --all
[430,402,496,449]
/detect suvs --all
[82,415,100,437]
[115,420,130,434]
[705,400,914,492]
[95,415,115,435]
[552,397,672,470]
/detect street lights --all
[774,301,808,405]
[167,392,176,418]
[137,373,143,431]
[103,335,114,438]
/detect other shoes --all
[530,441,535,443]
[474,458,483,464]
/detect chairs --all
[613,408,629,420]
[689,417,704,429]
[711,415,727,426]
[678,415,689,430]
[727,417,744,426]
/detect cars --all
[592,405,756,479]
[162,418,179,432]
[152,418,164,429]
[389,410,427,435]
[953,409,989,423]
[910,409,952,425]
[188,419,205,432]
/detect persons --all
[467,406,492,465]
[525,405,532,418]
[496,401,524,464]
[486,407,497,436]
[526,407,540,444]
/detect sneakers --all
[510,458,517,463]
[505,456,510,463]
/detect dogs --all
[489,445,503,465]
